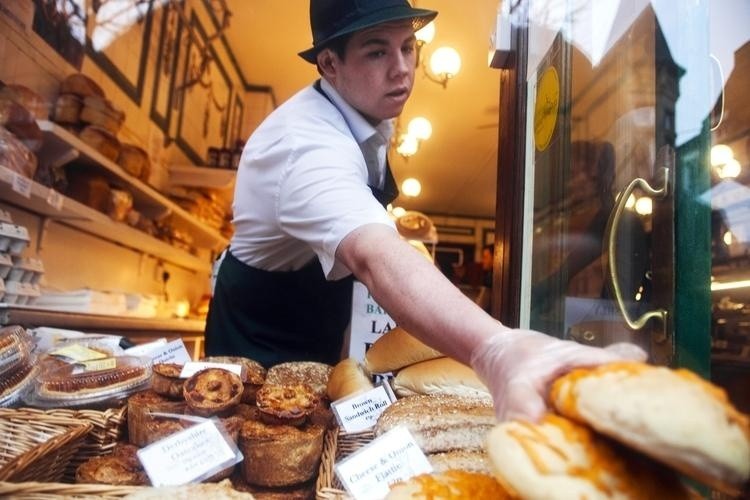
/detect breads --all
[395,209,434,241]
[0,321,749,499]
[1,73,235,268]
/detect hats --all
[297,0,439,64]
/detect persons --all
[534,207,652,320]
[475,244,494,310]
[202,0,649,425]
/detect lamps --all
[385,0,460,219]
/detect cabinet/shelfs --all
[0,304,207,364]
